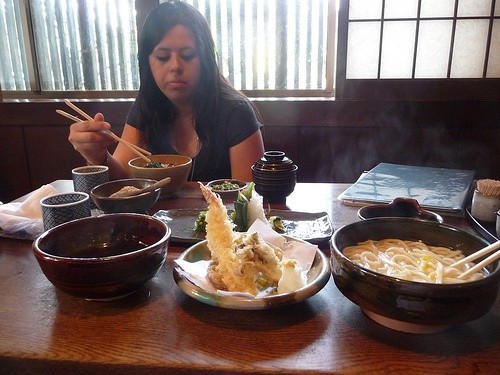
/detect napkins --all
[0,184,58,230]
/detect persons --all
[69,1,265,183]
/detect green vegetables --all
[195,212,208,232]
[143,162,163,169]
[212,182,241,190]
[230,183,254,231]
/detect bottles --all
[250,151,298,202]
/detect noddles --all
[341,238,489,284]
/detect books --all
[340,163,476,217]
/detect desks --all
[0,179,500,375]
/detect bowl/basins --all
[90,177,162,214]
[32,213,172,303]
[127,153,193,199]
[330,216,500,335]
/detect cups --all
[355,196,443,223]
[72,165,109,195]
[39,191,91,231]
[471,189,500,221]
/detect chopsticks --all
[449,240,500,280]
[56,100,151,163]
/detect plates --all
[151,208,335,246]
[173,232,332,310]
[206,179,248,197]
